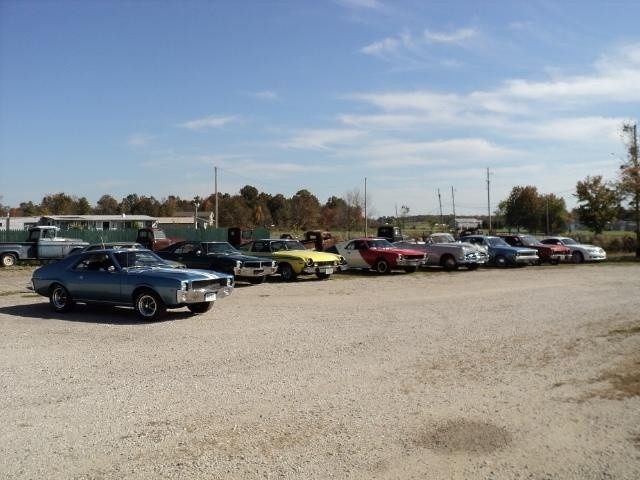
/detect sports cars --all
[31,248,235,323]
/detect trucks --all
[369,225,409,244]
[299,230,341,252]
[227,227,256,249]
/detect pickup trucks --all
[0,225,90,266]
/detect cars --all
[136,227,186,253]
[64,242,186,269]
[153,241,279,287]
[496,234,573,267]
[457,234,540,268]
[235,239,345,282]
[324,238,427,275]
[540,236,607,265]
[387,232,490,271]
